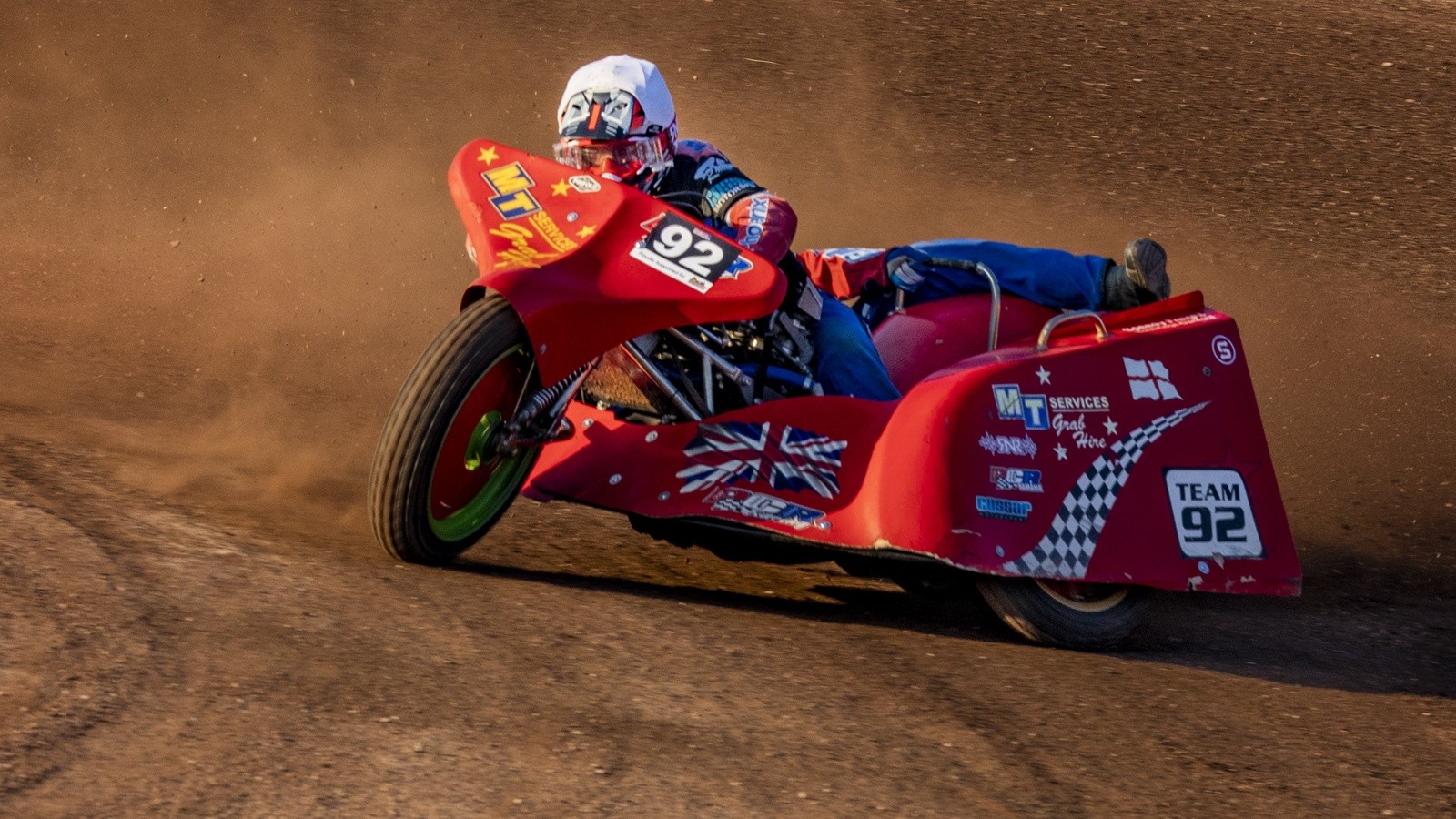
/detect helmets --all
[557,54,679,196]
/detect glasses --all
[552,136,663,181]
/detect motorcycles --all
[365,136,1306,657]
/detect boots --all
[1096,237,1170,311]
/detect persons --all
[549,53,899,425]
[764,231,1176,318]
